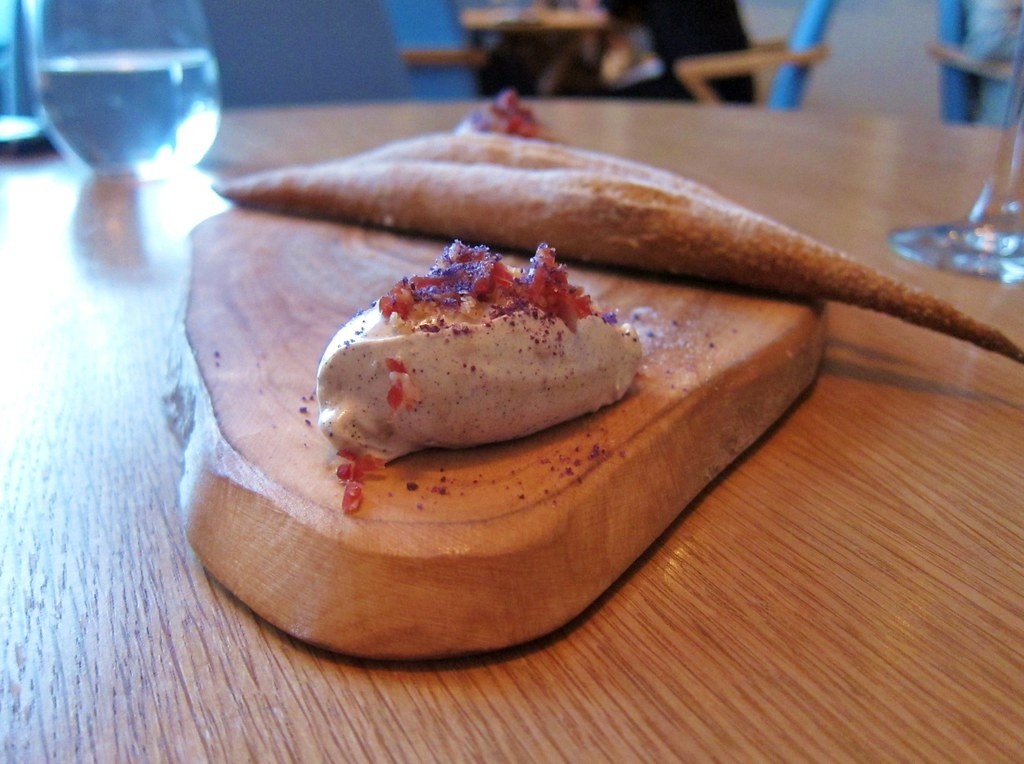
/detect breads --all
[210,135,1024,365]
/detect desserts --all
[312,239,641,470]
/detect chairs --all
[673,37,832,106]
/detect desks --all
[0,99,1024,764]
[460,8,612,63]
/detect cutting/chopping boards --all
[173,130,829,666]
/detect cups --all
[28,0,220,179]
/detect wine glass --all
[890,0,1024,280]
